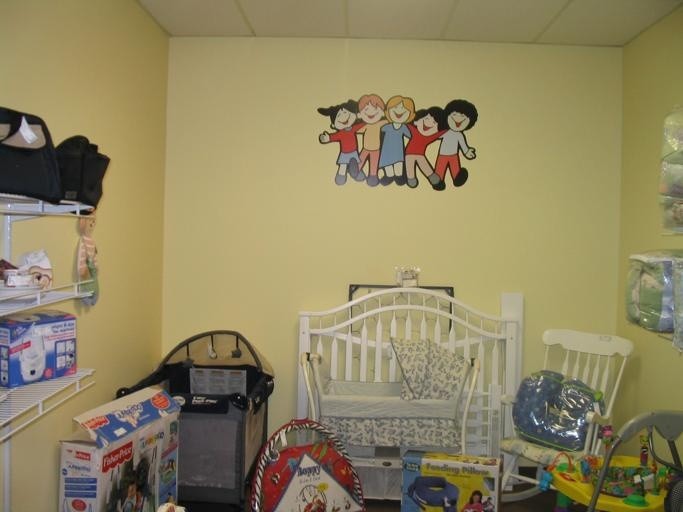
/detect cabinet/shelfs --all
[0,188,97,441]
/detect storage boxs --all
[53,385,181,512]
[0,309,78,389]
[401,450,503,511]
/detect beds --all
[290,281,527,503]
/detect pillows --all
[389,337,429,398]
[397,377,413,400]
[419,340,470,405]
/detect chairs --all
[498,328,636,512]
[586,406,682,511]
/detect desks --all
[551,453,668,511]
